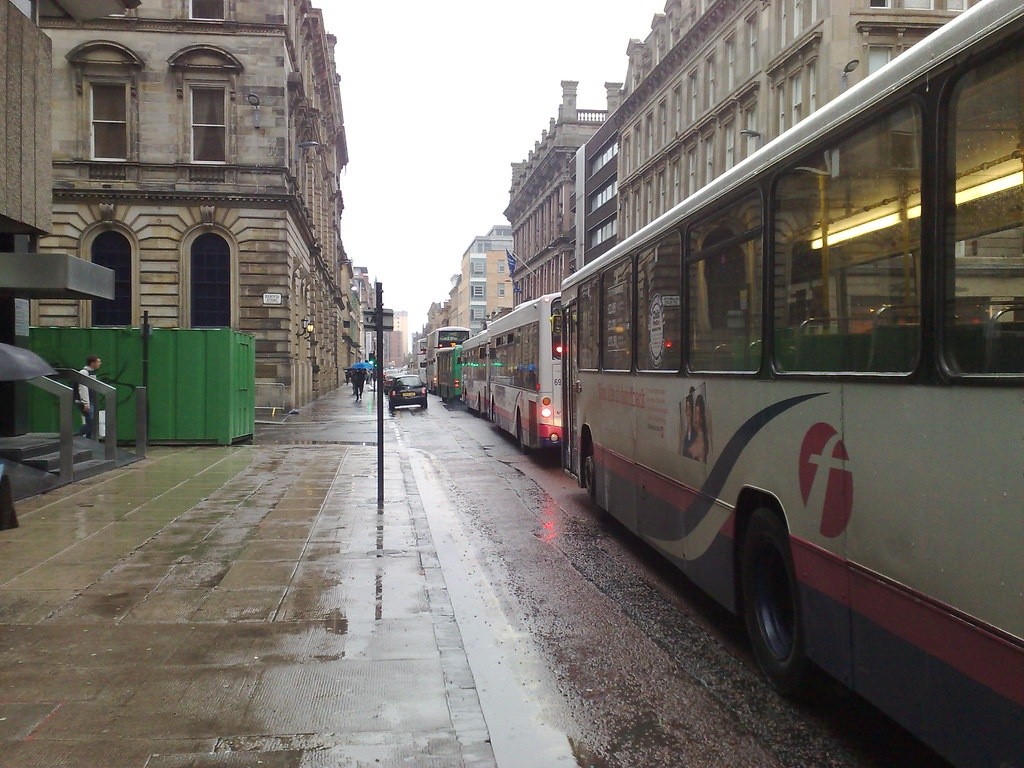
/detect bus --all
[420,326,472,396]
[478,292,560,456]
[457,327,491,420]
[410,338,429,385]
[550,1,1024,768]
[432,345,463,403]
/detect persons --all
[75,355,101,440]
[345,369,370,400]
[683,395,708,463]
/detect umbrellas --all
[0,343,58,382]
[343,362,373,370]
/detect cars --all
[385,374,430,409]
[384,363,408,396]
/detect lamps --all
[304,320,315,340]
[248,93,261,129]
[296,315,309,336]
